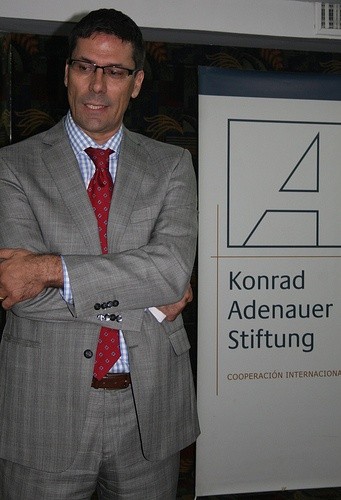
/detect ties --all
[85,148,122,379]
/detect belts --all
[91,372,131,390]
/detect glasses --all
[67,53,139,80]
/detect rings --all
[0,297,5,300]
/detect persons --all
[0,8,199,500]
